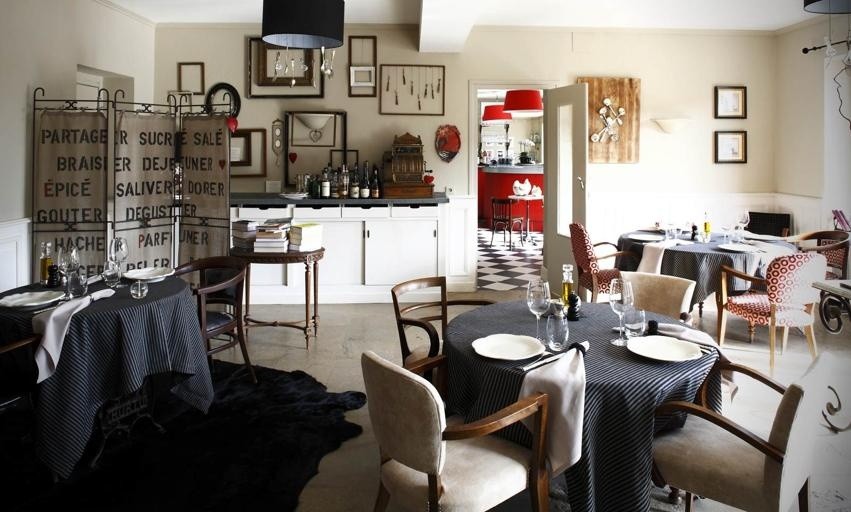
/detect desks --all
[615,225,797,343]
[3,276,214,479]
[508,193,545,249]
[440,300,733,508]
[229,247,325,350]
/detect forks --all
[516,351,557,369]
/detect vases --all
[520,152,533,164]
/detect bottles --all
[38,240,60,288]
[478,150,514,166]
[296,160,382,199]
[552,264,582,321]
[666,212,712,244]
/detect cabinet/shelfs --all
[230,191,453,304]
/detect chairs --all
[488,194,525,253]
[718,252,827,371]
[171,256,257,394]
[653,362,830,511]
[794,229,849,281]
[360,350,550,512]
[391,276,500,388]
[619,271,695,326]
[1,327,43,452]
[568,223,635,304]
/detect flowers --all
[516,140,538,154]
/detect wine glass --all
[527,278,552,347]
[58,246,89,300]
[720,210,752,245]
[110,236,130,289]
[609,278,635,348]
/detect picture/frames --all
[175,33,463,184]
[712,83,749,122]
[712,129,750,165]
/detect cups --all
[480,133,510,144]
[546,314,570,352]
[623,306,646,338]
[519,138,536,148]
[103,261,122,289]
[130,280,150,301]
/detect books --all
[232,217,322,253]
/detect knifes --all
[522,352,567,374]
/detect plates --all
[629,233,665,243]
[122,266,177,284]
[717,243,761,254]
[627,335,704,363]
[471,333,547,362]
[0,290,66,313]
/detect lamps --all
[800,0,851,66]
[482,93,517,125]
[502,88,546,117]
[260,0,348,88]
